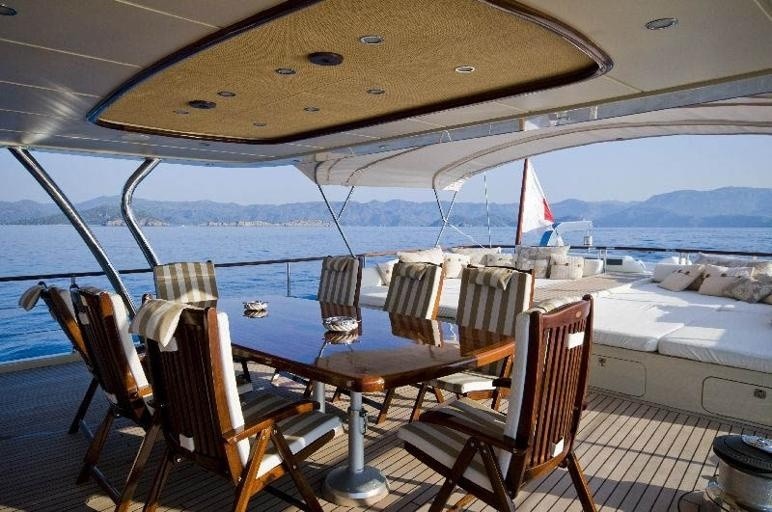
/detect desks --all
[214,295,515,507]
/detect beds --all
[350,256,772,428]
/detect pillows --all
[652,252,772,306]
[376,245,604,286]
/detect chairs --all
[409,264,536,422]
[67,282,253,512]
[330,259,446,425]
[394,294,597,512]
[271,254,364,401]
[128,293,340,512]
[153,260,251,386]
[16,282,147,475]
